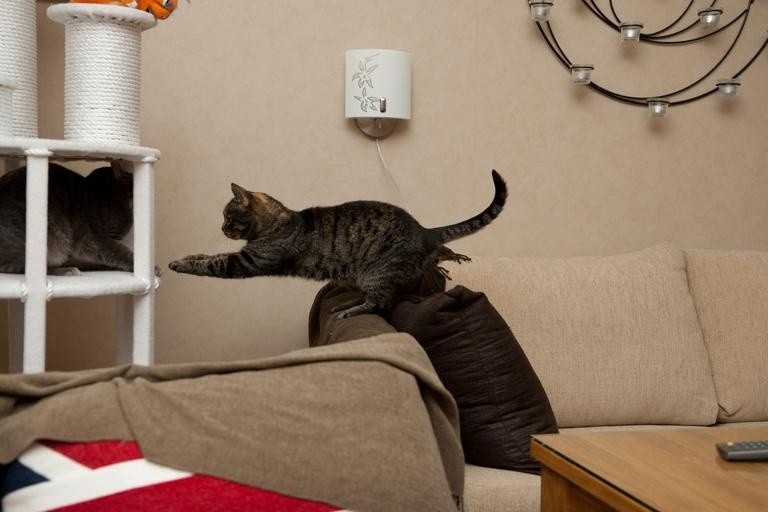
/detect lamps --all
[340,47,414,140]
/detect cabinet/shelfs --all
[0,133,161,378]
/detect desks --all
[528,424,768,512]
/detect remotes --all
[716,440,768,460]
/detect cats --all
[0,159,164,277]
[167,169,510,321]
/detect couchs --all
[309,241,767,512]
